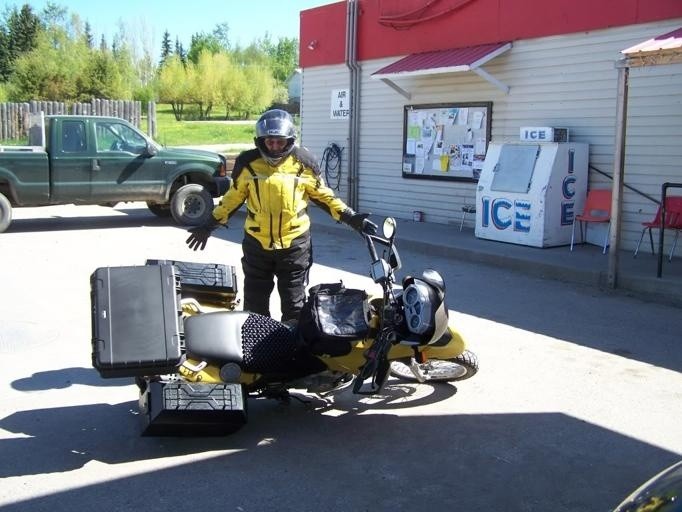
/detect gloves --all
[340,207,379,241]
[185,213,220,251]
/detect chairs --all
[632,196,682,264]
[569,189,613,254]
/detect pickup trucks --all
[0,112,231,229]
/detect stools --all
[459,202,476,230]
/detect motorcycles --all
[82,216,480,436]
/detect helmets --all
[253,109,297,168]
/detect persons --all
[186,109,378,323]
